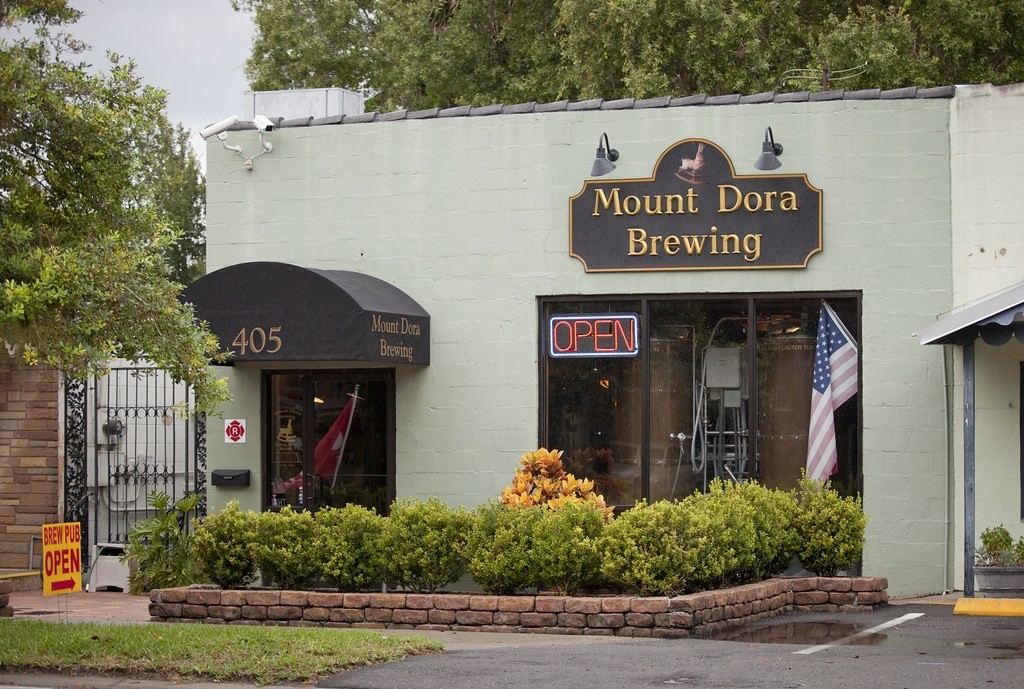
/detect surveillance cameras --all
[199,116,242,154]
[252,115,274,152]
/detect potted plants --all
[972,525,1024,599]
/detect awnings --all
[175,259,430,366]
[912,278,1024,348]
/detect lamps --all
[754,126,784,170]
[590,132,619,177]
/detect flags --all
[802,297,860,490]
[263,391,357,496]
